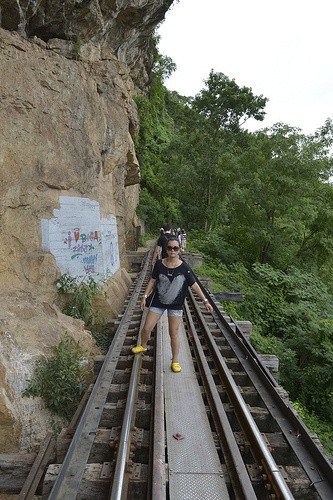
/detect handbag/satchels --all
[145,289,156,307]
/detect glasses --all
[166,245,181,252]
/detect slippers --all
[132,345,147,353]
[171,362,182,372]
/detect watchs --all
[142,294,148,299]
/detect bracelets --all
[203,299,209,305]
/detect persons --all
[159,226,188,253]
[155,223,178,266]
[131,236,213,373]
